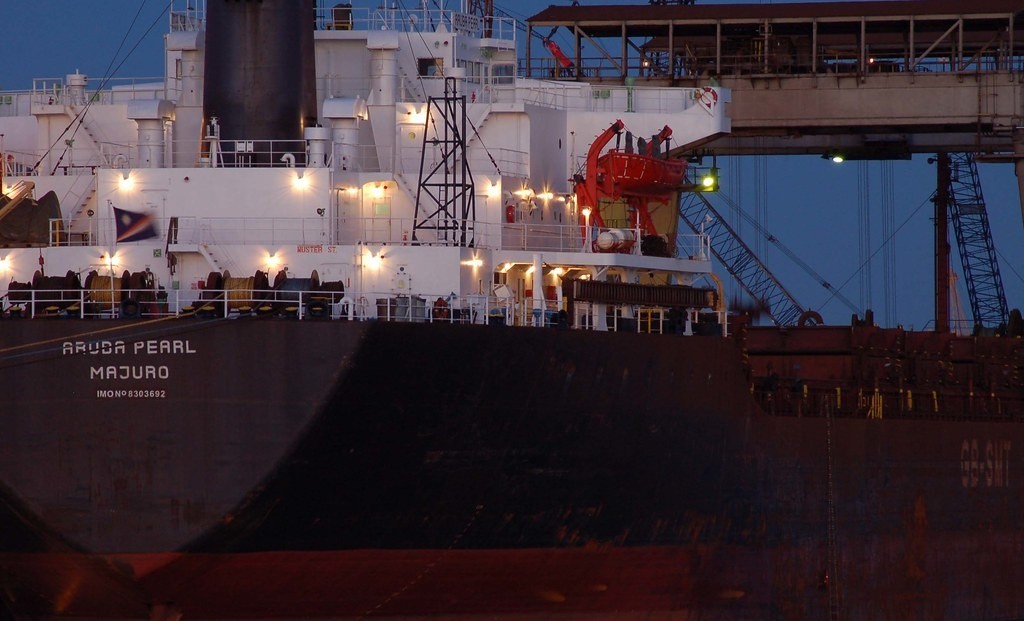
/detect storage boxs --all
[743,326,1024,415]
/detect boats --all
[598,131,689,192]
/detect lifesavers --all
[434,298,448,319]
[698,87,718,107]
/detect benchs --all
[58,166,100,176]
[326,24,353,30]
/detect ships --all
[0,0,1023,583]
